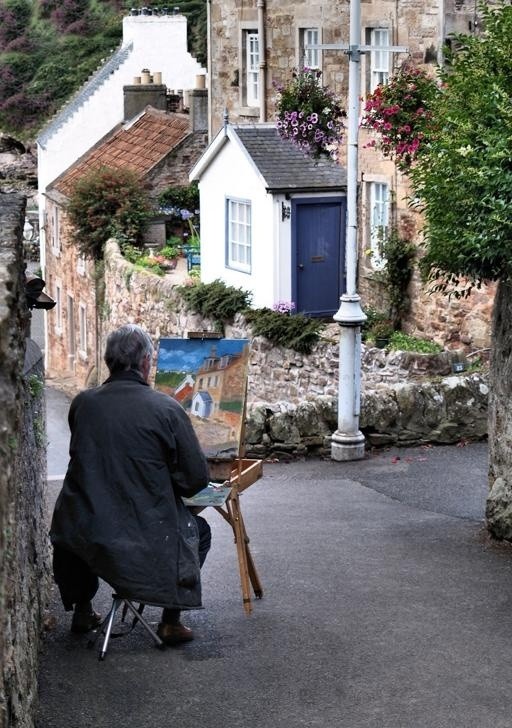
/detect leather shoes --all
[72,609,101,636]
[157,622,195,642]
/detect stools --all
[85,593,168,660]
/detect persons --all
[45,321,213,648]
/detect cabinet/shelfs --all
[120,330,267,630]
